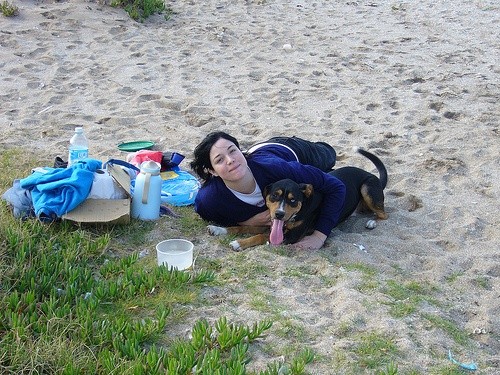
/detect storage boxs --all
[62,164,131,225]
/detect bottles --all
[67,127,89,165]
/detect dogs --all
[206,147,389,253]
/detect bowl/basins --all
[156,239,194,271]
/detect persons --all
[189,131,348,250]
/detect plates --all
[117,141,154,151]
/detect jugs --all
[131,160,162,219]
